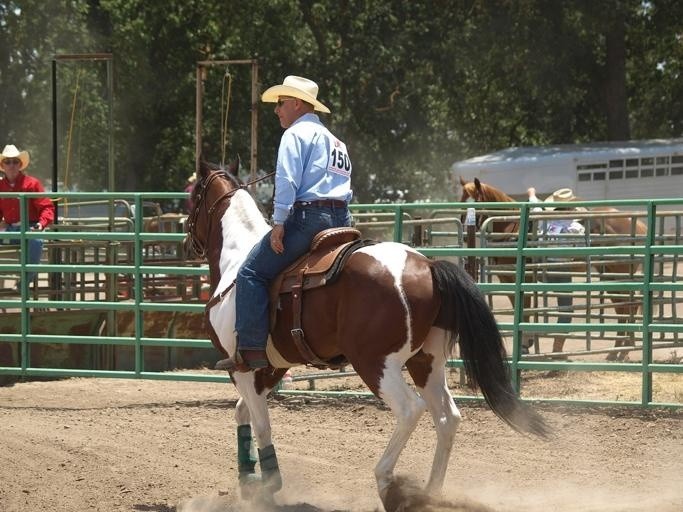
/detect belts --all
[295,199,347,208]
[12,222,21,227]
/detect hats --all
[0,144,30,171]
[544,188,576,212]
[261,76,331,114]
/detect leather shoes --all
[214,350,268,371]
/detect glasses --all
[3,159,21,165]
[277,99,303,107]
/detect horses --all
[179,152,554,512]
[458,174,649,364]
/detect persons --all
[517,186,593,363]
[214,76,356,370]
[0,144,54,300]
[186,171,197,215]
